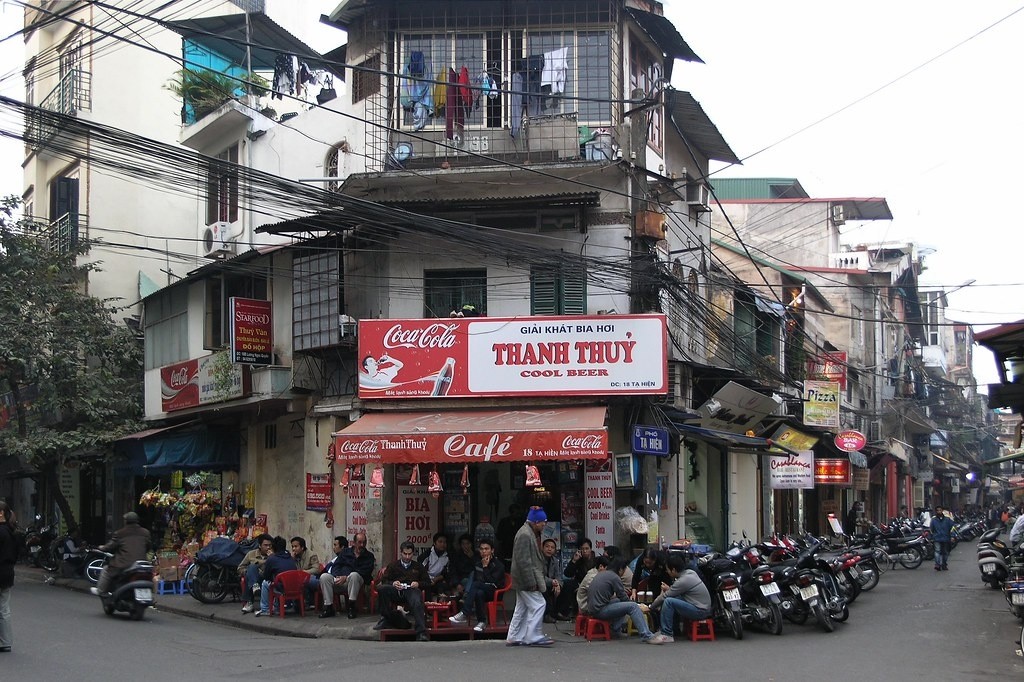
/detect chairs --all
[242,565,512,629]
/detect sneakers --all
[449,611,467,622]
[646,634,663,643]
[241,602,253,612]
[90,587,108,596]
[533,635,554,644]
[252,583,261,595]
[474,621,487,631]
[0,646,11,652]
[934,565,941,571]
[655,631,675,642]
[942,565,948,570]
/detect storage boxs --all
[157,555,197,581]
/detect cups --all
[646,591,654,603]
[637,591,645,602]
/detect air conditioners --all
[686,180,709,212]
[200,220,234,260]
[833,202,846,228]
[652,361,694,413]
[868,419,883,443]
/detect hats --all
[527,506,548,523]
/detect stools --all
[157,581,176,596]
[686,619,714,642]
[583,618,610,642]
[177,578,193,594]
[576,615,593,639]
[627,612,655,636]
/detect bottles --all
[375,355,388,365]
[430,356,456,396]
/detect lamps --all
[694,379,722,417]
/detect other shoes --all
[319,611,335,617]
[555,612,571,621]
[416,631,431,641]
[543,614,556,623]
[373,618,390,629]
[346,605,357,618]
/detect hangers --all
[480,62,508,77]
[222,56,248,72]
[184,43,207,56]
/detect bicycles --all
[181,533,264,602]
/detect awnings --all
[326,403,612,461]
[112,417,242,478]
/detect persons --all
[237,504,714,650]
[0,501,18,653]
[62,523,90,576]
[90,511,154,600]
[843,501,1024,572]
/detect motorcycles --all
[687,500,1024,640]
[13,514,156,585]
[90,546,158,620]
